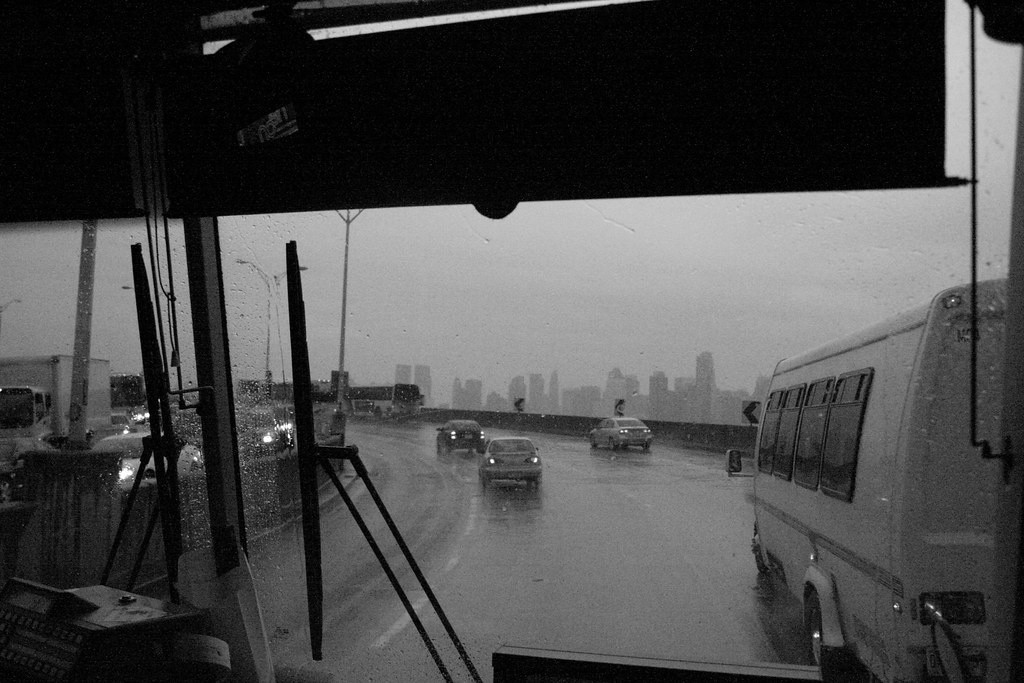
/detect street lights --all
[273,265,306,420]
[237,258,272,406]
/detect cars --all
[589,417,655,451]
[436,419,485,457]
[89,430,203,493]
[476,435,543,492]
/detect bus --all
[725,273,1024,683]
[1,385,52,437]
[321,383,421,425]
